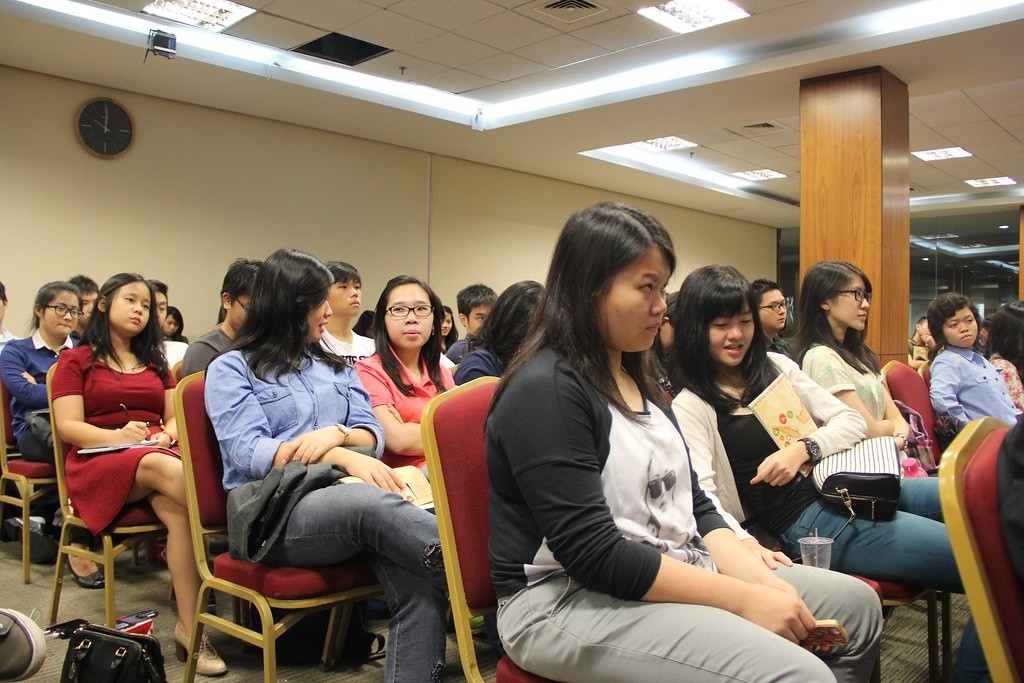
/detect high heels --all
[65,554,105,588]
[173,622,228,676]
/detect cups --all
[798,537,835,570]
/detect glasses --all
[836,290,872,304]
[385,305,435,317]
[760,302,790,310]
[46,305,84,321]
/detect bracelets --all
[893,433,908,451]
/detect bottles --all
[901,456,930,480]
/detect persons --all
[669,264,993,683]
[204,249,453,683]
[52,272,227,675]
[928,293,1022,430]
[788,260,945,524]
[485,201,883,683]
[0,259,1024,683]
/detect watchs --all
[164,431,177,447]
[797,437,822,464]
[334,424,350,445]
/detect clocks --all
[73,96,137,160]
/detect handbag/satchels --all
[933,416,959,453]
[813,436,902,522]
[42,618,167,683]
[333,465,437,514]
[892,399,936,473]
[24,408,53,449]
[250,601,385,662]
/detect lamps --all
[144,29,177,64]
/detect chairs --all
[0,357,1024,683]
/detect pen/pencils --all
[119,403,149,441]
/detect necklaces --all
[714,381,746,396]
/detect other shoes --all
[0,608,46,683]
[469,615,486,634]
